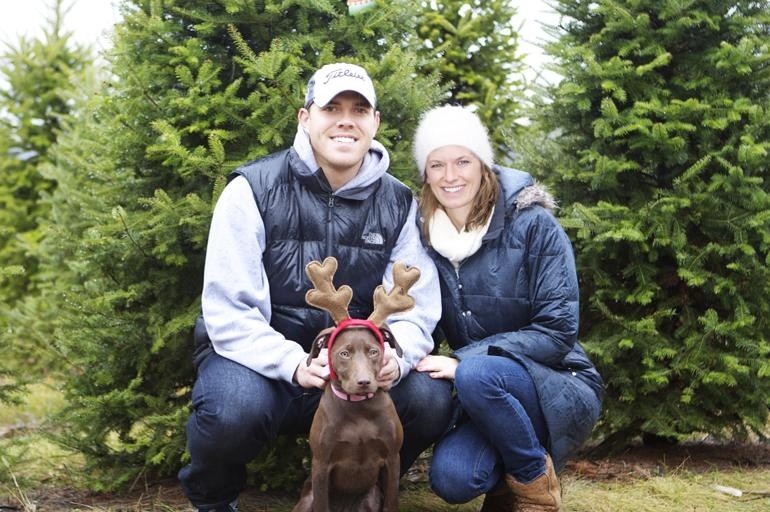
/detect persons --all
[177,62,443,512]
[411,102,606,511]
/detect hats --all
[304,63,377,110]
[413,104,493,178]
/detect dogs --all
[293,323,404,512]
